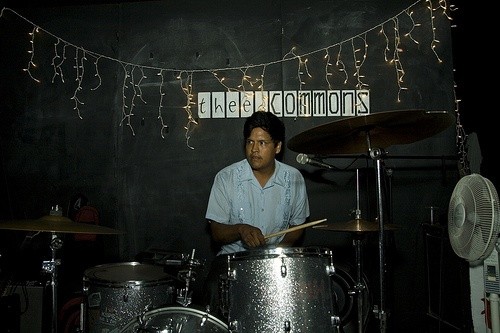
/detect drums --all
[330,260,370,327]
[415,204,448,229]
[112,303,229,333]
[212,244,343,333]
[81,260,174,333]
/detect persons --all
[204,111,310,259]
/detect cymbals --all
[286,108,453,157]
[309,219,404,234]
[1,215,127,237]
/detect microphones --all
[295,154,333,169]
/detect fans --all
[447,173,500,333]
